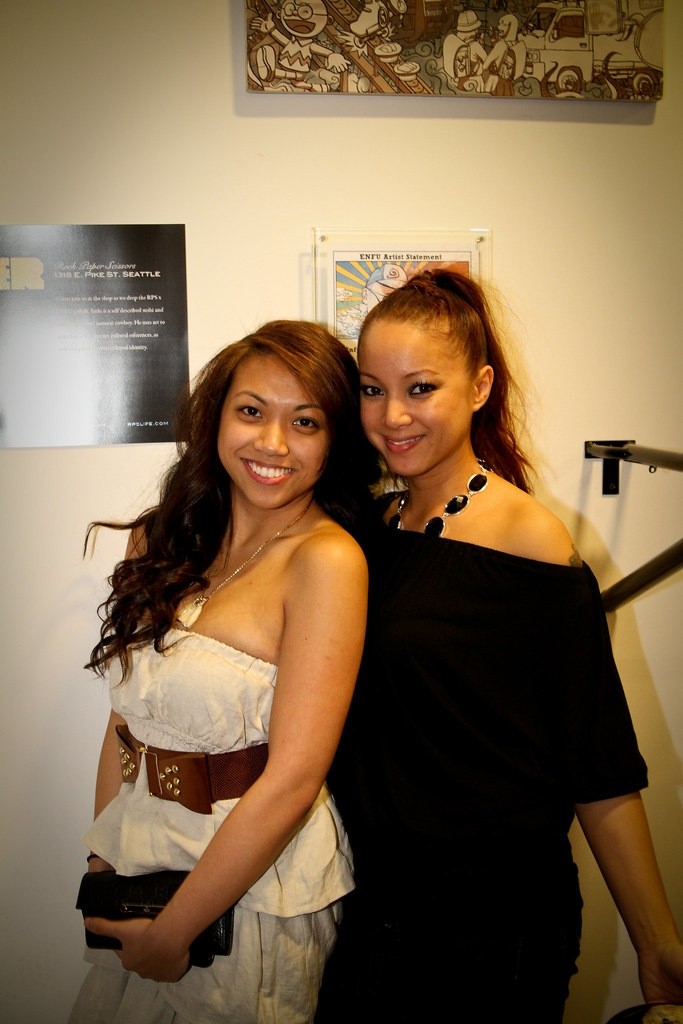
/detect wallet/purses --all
[75,870,236,968]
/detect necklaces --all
[177,499,310,629]
[390,458,500,536]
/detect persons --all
[62,318,369,1024]
[322,272,683,1023]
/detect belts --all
[112,722,265,814]
